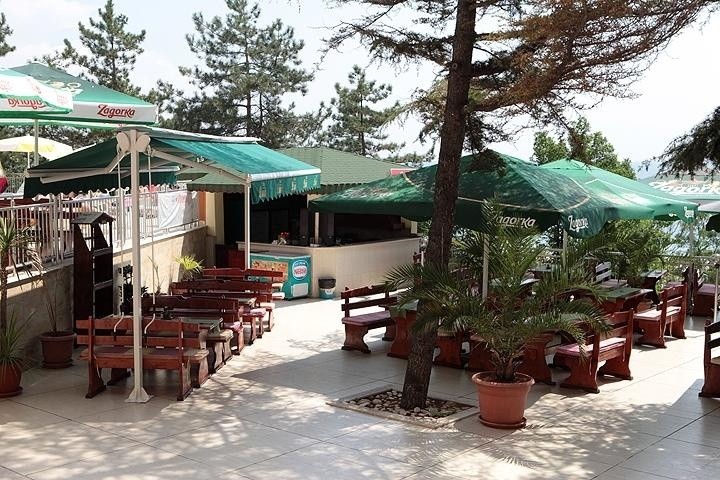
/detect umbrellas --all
[0,133,74,169]
[0,60,162,161]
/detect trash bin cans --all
[318,278,337,299]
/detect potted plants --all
[0,219,77,399]
[379,189,613,429]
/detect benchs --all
[74,269,285,400]
[340,262,720,398]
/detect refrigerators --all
[249,251,310,300]
